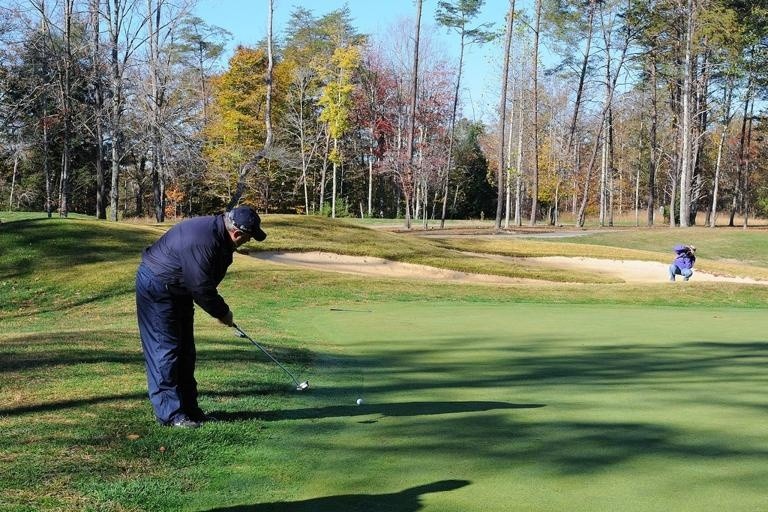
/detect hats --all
[226,206,266,241]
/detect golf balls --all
[356,399,362,405]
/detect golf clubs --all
[233,323,308,390]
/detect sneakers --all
[172,416,200,428]
[191,409,216,422]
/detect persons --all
[668,244,696,281]
[135,205,267,429]
